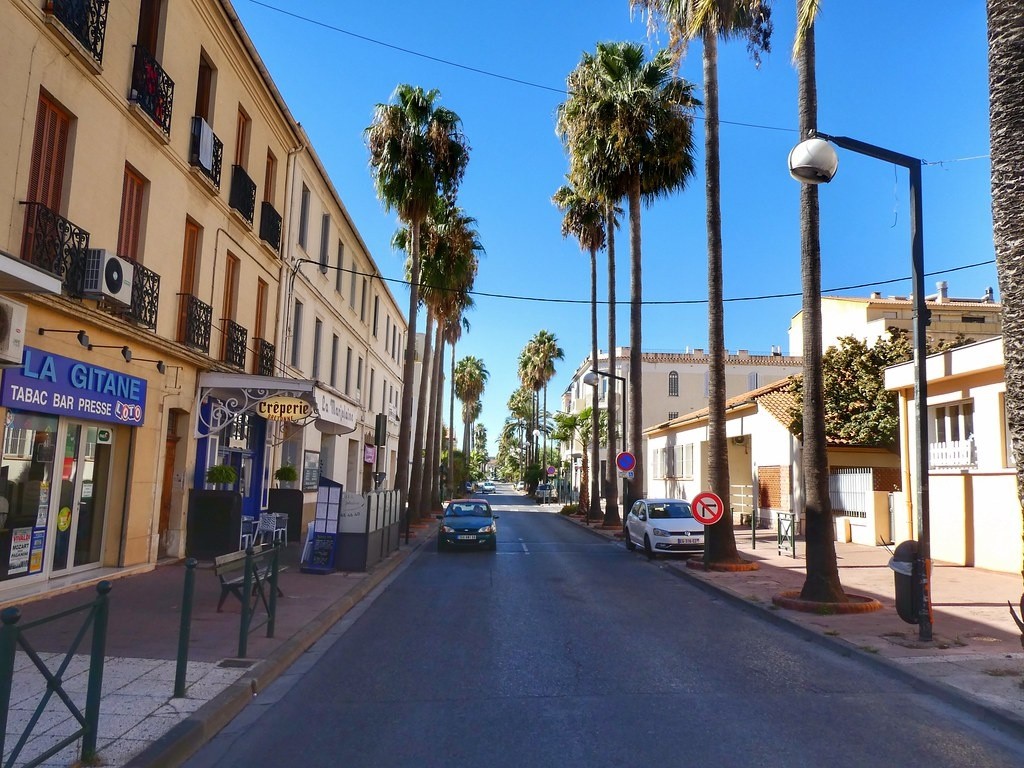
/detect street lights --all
[789,128,934,643]
[582,369,631,537]
[533,427,553,504]
[526,441,541,451]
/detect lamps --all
[38,327,89,347]
[88,343,132,363]
[131,358,165,375]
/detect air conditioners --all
[83,250,135,306]
[0,294,28,365]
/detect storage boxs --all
[0,480,51,579]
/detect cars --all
[481,482,496,494]
[535,483,559,503]
[466,482,472,495]
[436,498,499,551]
[516,481,528,491]
[625,499,706,561]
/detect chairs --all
[240,512,289,550]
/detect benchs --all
[215,544,283,615]
